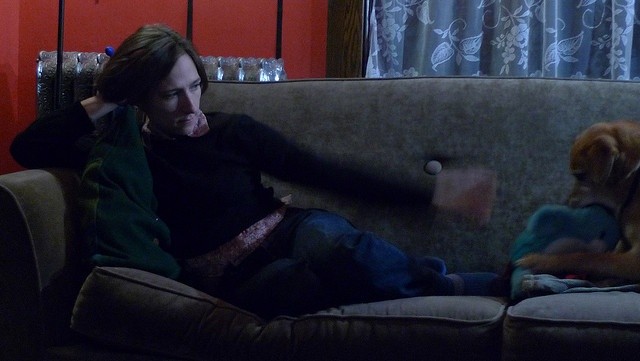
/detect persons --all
[9,22,508,319]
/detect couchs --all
[0,77,639,360]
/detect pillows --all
[81,103,187,287]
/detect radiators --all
[33,50,289,123]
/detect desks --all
[9,112,94,175]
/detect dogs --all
[513,121,640,284]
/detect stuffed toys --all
[506,201,623,303]
